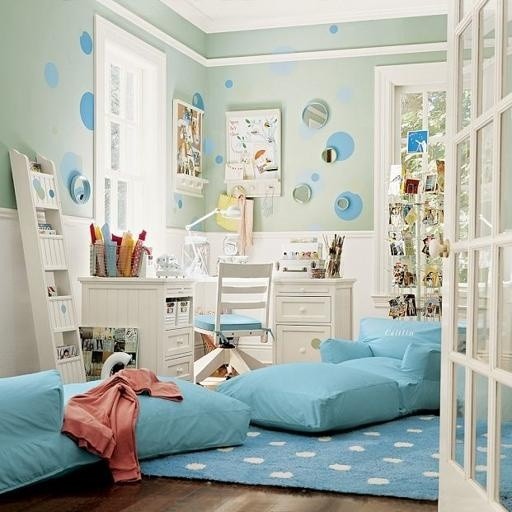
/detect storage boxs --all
[176,301,190,325]
[166,302,176,328]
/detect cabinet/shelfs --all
[9,149,86,384]
[273,278,355,364]
[77,276,193,381]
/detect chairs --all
[194,261,272,382]
[216,318,465,432]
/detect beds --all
[0,369,251,496]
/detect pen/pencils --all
[328,233,346,254]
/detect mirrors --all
[321,148,338,164]
[337,198,349,210]
[302,103,328,129]
[292,184,311,204]
[71,176,91,204]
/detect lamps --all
[186,205,241,276]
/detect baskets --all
[88,242,148,279]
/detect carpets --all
[139,414,512,512]
